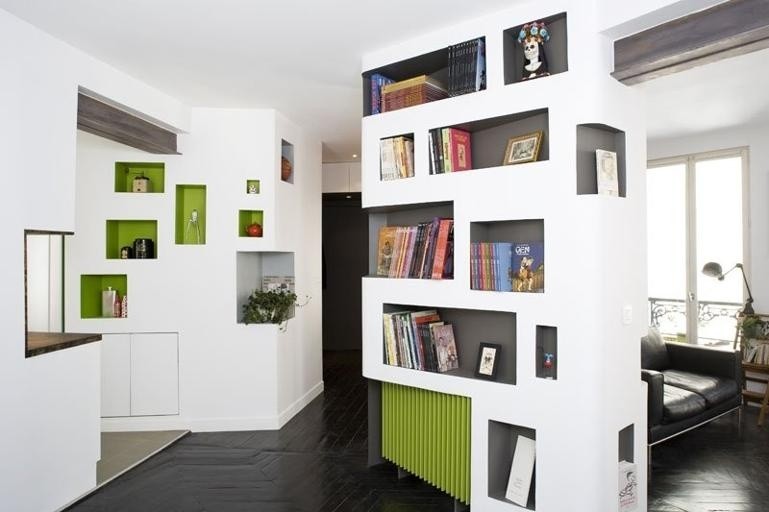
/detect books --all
[746,343,769,365]
[368,40,482,115]
[504,434,536,507]
[377,217,453,280]
[382,311,461,372]
[427,130,473,174]
[470,243,545,292]
[379,136,413,181]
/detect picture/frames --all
[474,342,501,379]
[503,130,544,165]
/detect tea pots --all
[245,221,263,237]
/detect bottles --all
[183,209,200,245]
[543,353,555,379]
[101,286,127,318]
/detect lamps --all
[702,263,755,314]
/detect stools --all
[741,360,769,426]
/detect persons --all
[595,149,619,197]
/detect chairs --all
[641,330,744,485]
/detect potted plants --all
[243,285,297,325]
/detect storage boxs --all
[380,76,448,112]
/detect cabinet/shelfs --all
[361,4,648,512]
[63,107,324,431]
